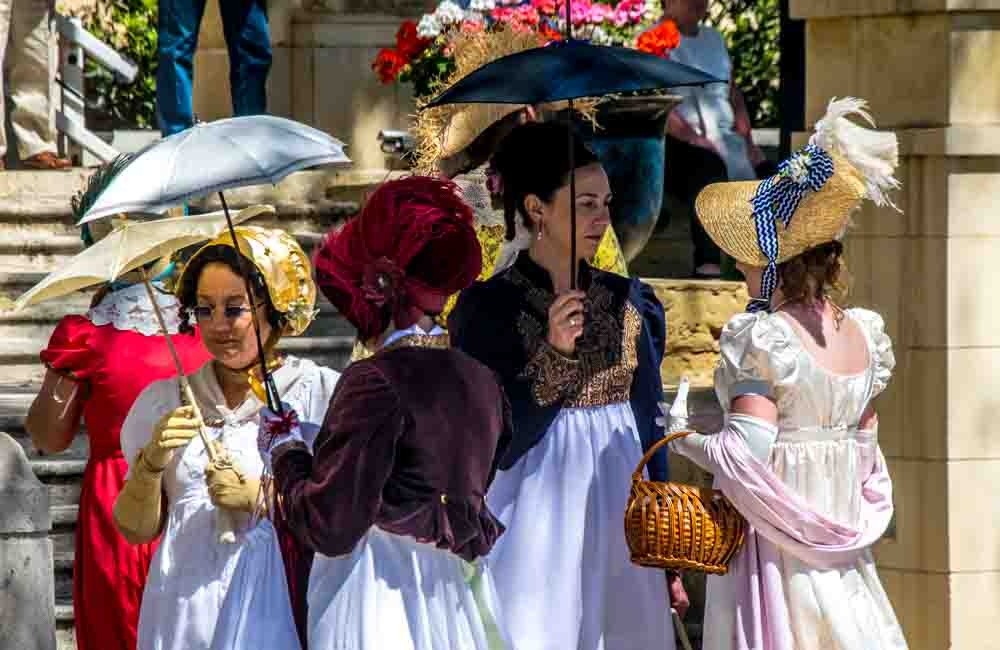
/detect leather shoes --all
[24,150,72,172]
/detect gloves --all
[257,401,305,454]
[111,405,203,544]
[204,460,286,518]
[658,377,779,476]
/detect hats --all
[314,176,482,336]
[695,96,900,314]
[177,226,317,337]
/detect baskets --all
[621,432,747,575]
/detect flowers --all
[370,0,681,97]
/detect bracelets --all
[136,447,167,473]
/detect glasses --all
[189,302,264,322]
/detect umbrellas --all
[74,114,354,412]
[424,38,729,293]
[15,206,278,465]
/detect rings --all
[569,316,578,327]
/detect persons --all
[26,253,297,650]
[0,0,72,170]
[110,228,343,650]
[447,121,690,650]
[656,150,910,650]
[657,0,758,278]
[258,176,513,650]
[156,0,274,138]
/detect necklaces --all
[772,293,845,330]
